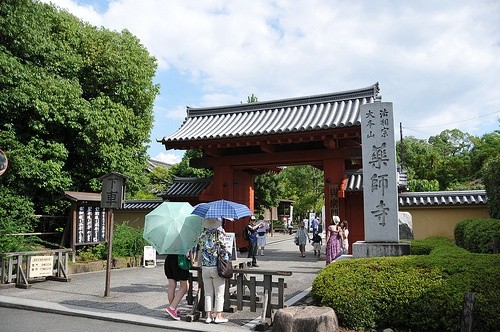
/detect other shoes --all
[260,253,265,256]
[256,253,259,256]
[317,254,320,258]
[213,316,228,323]
[252,262,259,267]
[298,253,302,257]
[302,255,306,258]
[205,317,213,324]
[314,252,317,256]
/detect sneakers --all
[175,310,181,315]
[166,306,180,321]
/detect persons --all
[164,244,197,320]
[326,215,344,267]
[255,215,268,256]
[296,225,310,258]
[246,216,265,267]
[339,221,349,256]
[312,216,320,237]
[312,232,323,258]
[282,220,288,235]
[198,218,229,323]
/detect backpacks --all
[242,223,251,240]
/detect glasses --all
[251,219,255,221]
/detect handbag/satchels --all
[217,253,233,278]
[178,254,192,270]
[258,232,265,236]
[336,226,342,239]
[296,240,299,245]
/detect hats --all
[298,221,305,227]
[332,215,340,225]
[315,231,318,234]
[202,218,221,228]
[250,216,256,220]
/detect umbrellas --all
[191,199,253,219]
[142,201,204,255]
